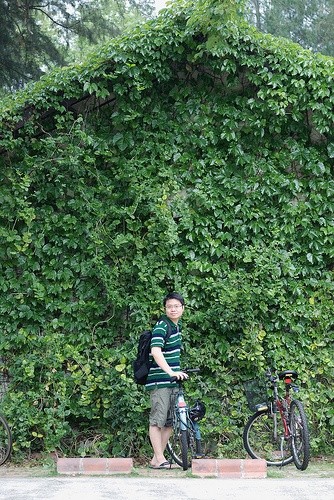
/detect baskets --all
[244,379,268,412]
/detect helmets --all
[189,402,206,422]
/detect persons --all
[146,293,189,469]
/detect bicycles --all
[241,356,310,471]
[164,367,203,472]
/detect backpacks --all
[134,318,170,384]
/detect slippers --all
[152,462,181,469]
[149,465,155,468]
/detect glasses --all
[163,305,183,310]
